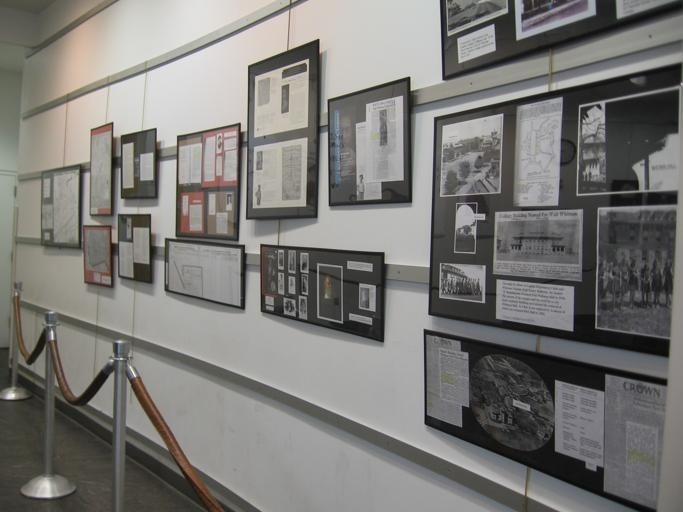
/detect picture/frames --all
[260,243,384,342]
[424,329,667,512]
[41,164,81,249]
[89,121,113,215]
[427,62,683,357]
[245,38,319,217]
[121,128,157,198]
[328,77,412,206]
[175,122,241,241]
[118,214,152,283]
[83,225,113,287]
[165,238,245,309]
[440,0,683,80]
